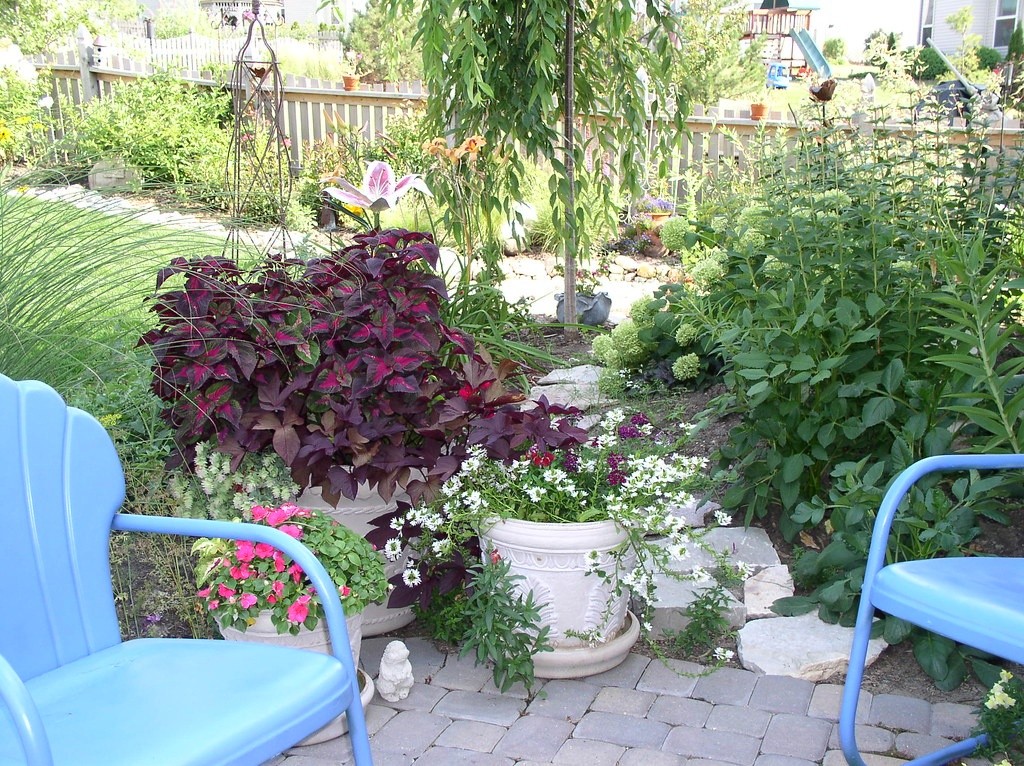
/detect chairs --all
[0,374,373,766]
[837,453,1024,766]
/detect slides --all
[788,25,836,82]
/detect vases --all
[342,76,360,90]
[468,500,640,648]
[243,439,456,637]
[636,213,670,258]
[205,595,362,678]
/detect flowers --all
[383,408,753,679]
[188,504,393,637]
[634,196,674,214]
[137,157,585,509]
[344,52,365,76]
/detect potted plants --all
[750,95,770,119]
